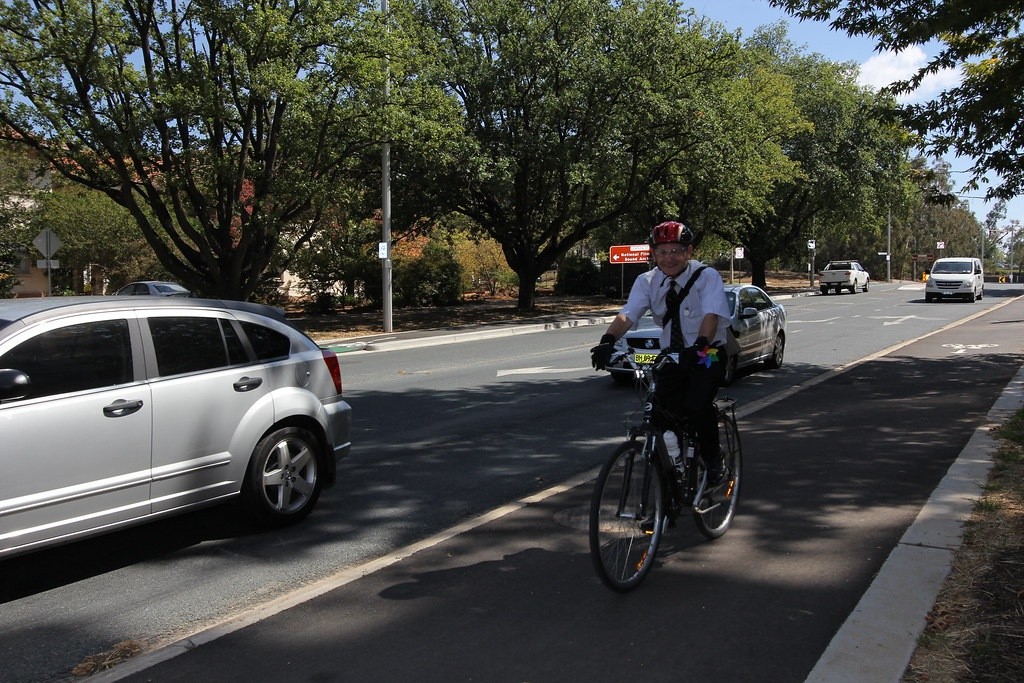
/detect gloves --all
[590,334,618,371]
[678,337,710,377]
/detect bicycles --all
[588,343,744,592]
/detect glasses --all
[653,247,687,259]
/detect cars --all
[111,280,192,300]
[597,284,787,386]
[0,297,357,567]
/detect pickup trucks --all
[816,259,871,294]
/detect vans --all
[925,257,985,303]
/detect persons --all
[590,220,731,532]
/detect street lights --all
[979,229,1006,273]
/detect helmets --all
[649,221,693,246]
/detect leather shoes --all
[706,444,727,486]
[641,506,677,530]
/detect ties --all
[666,281,685,349]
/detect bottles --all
[663,430,685,474]
[685,441,695,473]
